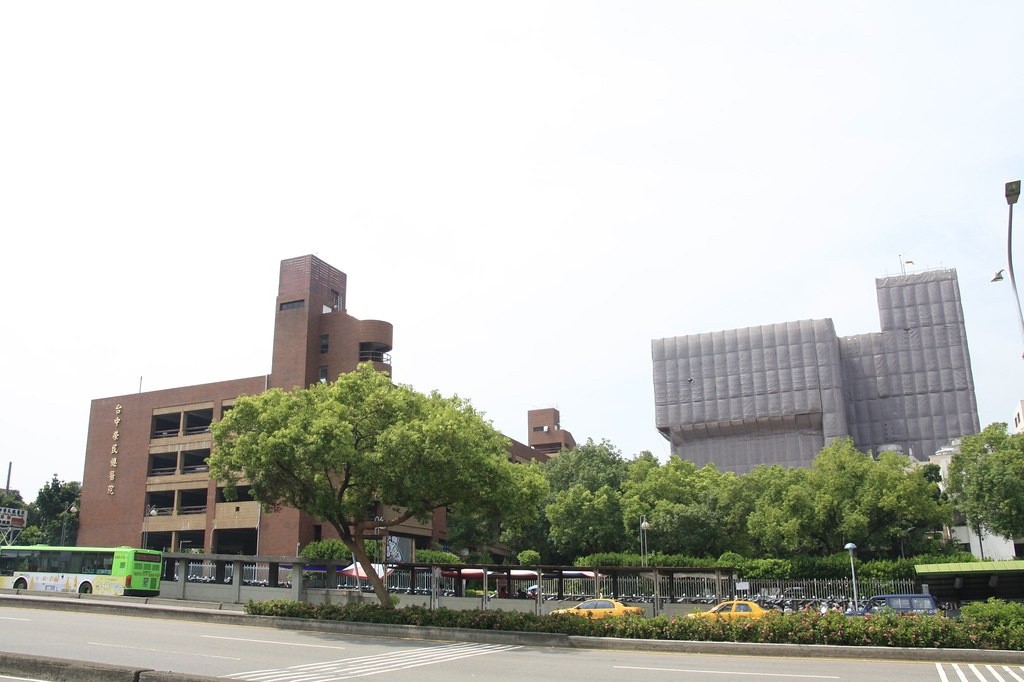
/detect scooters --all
[541,593,869,616]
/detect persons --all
[498,586,506,599]
[531,590,536,600]
[517,589,529,599]
[507,588,514,599]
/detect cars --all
[550,593,643,624]
[687,595,783,622]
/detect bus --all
[0,544,162,596]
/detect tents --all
[340,562,397,590]
[441,568,608,600]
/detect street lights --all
[142,505,157,549]
[60,501,77,546]
[639,514,650,566]
[992,179,1024,339]
[843,542,859,610]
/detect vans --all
[843,594,938,619]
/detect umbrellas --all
[528,585,544,591]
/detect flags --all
[901,257,913,263]
